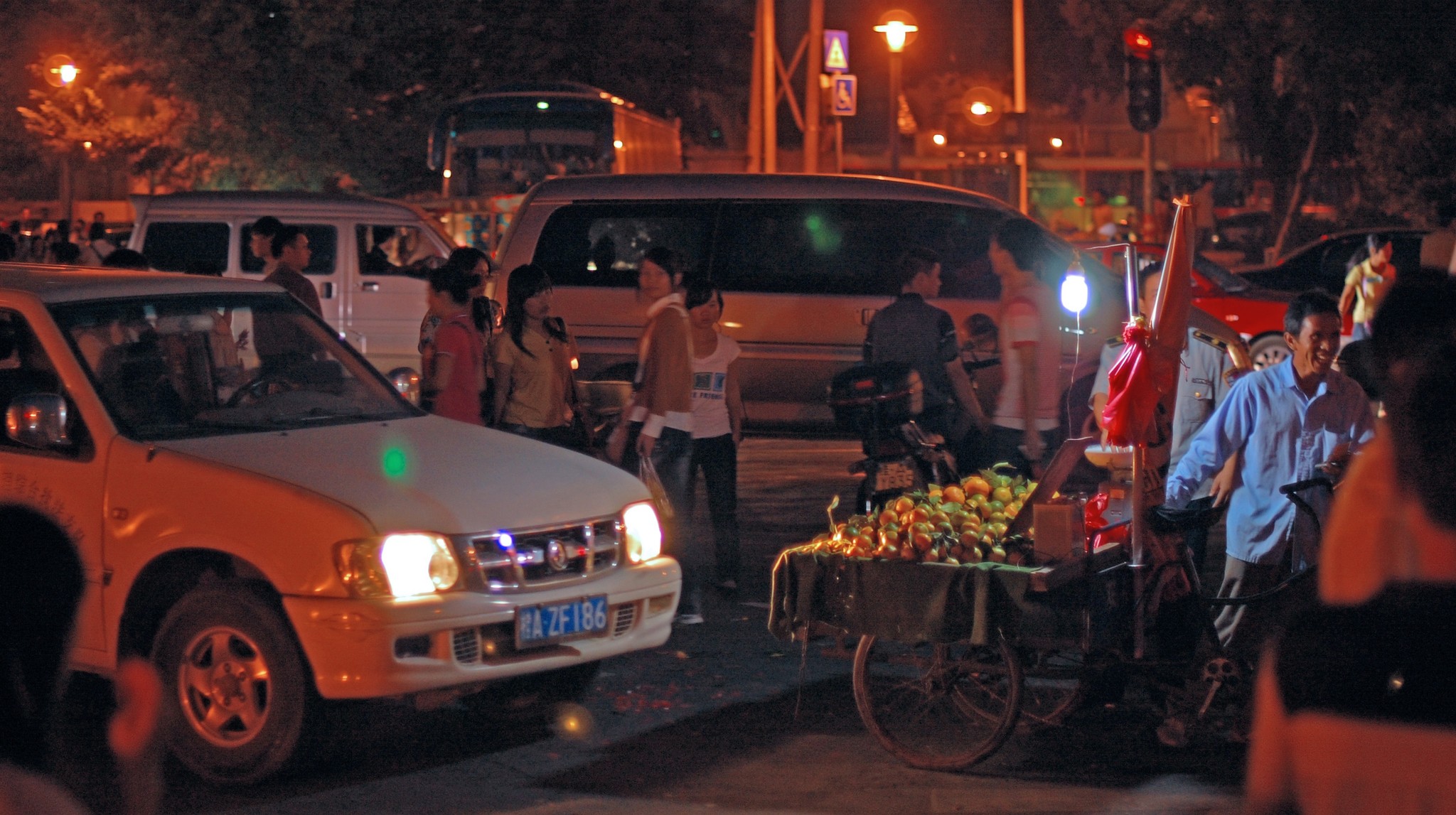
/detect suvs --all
[0,260,687,785]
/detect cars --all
[1091,245,1356,376]
[1227,228,1433,296]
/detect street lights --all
[49,64,82,223]
[875,17,918,177]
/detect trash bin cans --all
[566,380,634,458]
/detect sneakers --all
[673,599,704,624]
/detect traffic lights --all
[1123,26,1163,133]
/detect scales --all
[1085,445,1170,527]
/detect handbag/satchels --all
[605,398,636,464]
[556,406,594,447]
[1275,576,1456,732]
[637,455,676,554]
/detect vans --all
[126,194,461,392]
[480,172,1135,454]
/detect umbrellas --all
[1104,193,1194,514]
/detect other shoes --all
[1063,691,1122,736]
[1156,711,1202,744]
[711,571,750,596]
[1210,714,1243,745]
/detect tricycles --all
[781,472,1341,773]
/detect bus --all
[425,89,683,259]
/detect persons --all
[1156,290,1378,753]
[1339,231,1396,339]
[987,214,1062,478]
[678,279,744,591]
[0,207,324,405]
[495,264,591,451]
[1091,262,1238,692]
[860,248,989,474]
[420,248,501,430]
[1247,267,1456,815]
[606,246,704,624]
[361,225,397,275]
[419,267,487,427]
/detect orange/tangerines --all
[828,474,1065,568]
